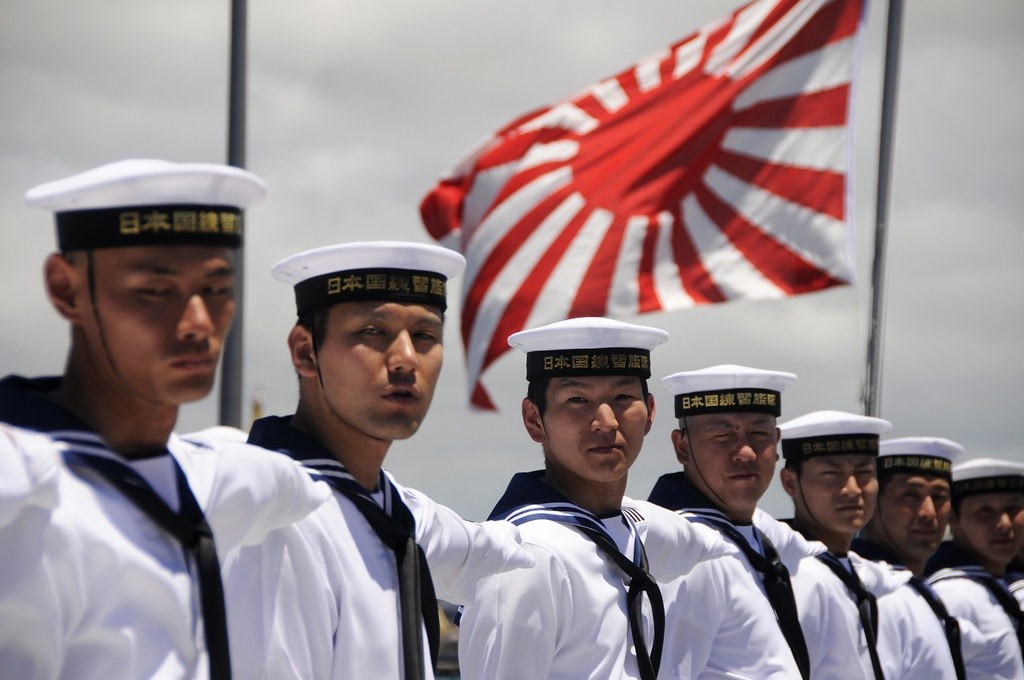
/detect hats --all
[506,316,670,378]
[19,156,266,256]
[876,437,965,478]
[775,410,893,459]
[660,365,800,419]
[948,457,1024,494]
[270,242,469,320]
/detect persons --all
[921,455,1024,680]
[852,436,988,680]
[0,154,334,680]
[625,365,831,679]
[774,407,915,679]
[448,315,744,680]
[172,239,547,680]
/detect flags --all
[418,0,867,413]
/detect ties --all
[980,577,1024,660]
[712,518,811,680]
[909,575,966,680]
[77,448,232,680]
[575,525,666,680]
[815,550,888,680]
[327,474,440,680]
